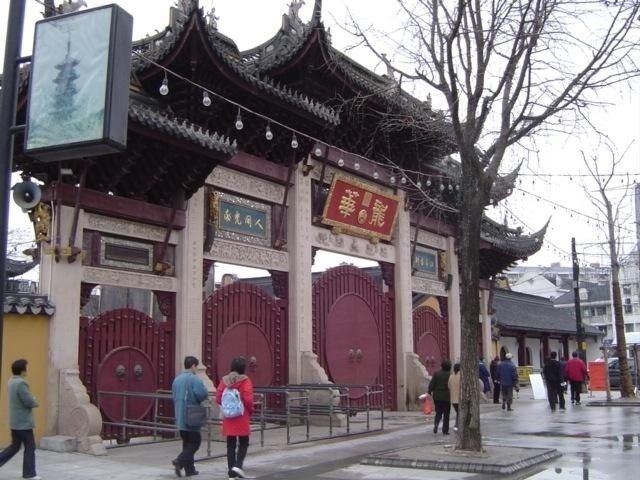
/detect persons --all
[559,357,567,394]
[447,363,461,431]
[543,351,566,413]
[496,353,520,412]
[0,359,40,478]
[173,355,208,476]
[216,357,256,480]
[489,355,503,404]
[541,355,558,403]
[427,360,452,435]
[566,351,587,404]
[479,356,490,394]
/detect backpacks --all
[221,372,245,419]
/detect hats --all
[504,353,513,359]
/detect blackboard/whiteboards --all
[529,374,547,399]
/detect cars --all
[584,357,640,388]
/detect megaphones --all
[13,181,43,210]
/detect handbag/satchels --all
[419,395,435,415]
[184,405,208,428]
[560,382,568,391]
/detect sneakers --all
[21,473,41,480]
[485,398,587,413]
[170,457,250,480]
[431,425,458,436]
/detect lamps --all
[490,176,636,267]
